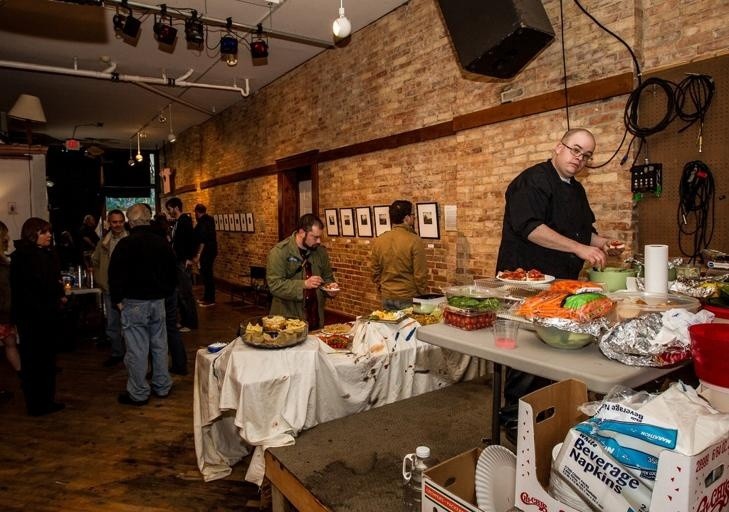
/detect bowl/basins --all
[688,322,729,389]
[587,267,634,292]
[603,291,701,323]
[532,317,593,352]
[239,315,309,348]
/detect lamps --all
[4,94,49,125]
[128,103,176,166]
[111,1,270,60]
[331,0,353,39]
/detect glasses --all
[561,142,593,162]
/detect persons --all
[1,217,65,418]
[266,213,338,332]
[72,197,218,405]
[494,126,624,446]
[369,199,433,315]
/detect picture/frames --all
[341,207,356,238]
[374,205,396,238]
[213,212,256,232]
[324,209,340,238]
[356,206,373,238]
[417,203,441,240]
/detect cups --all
[492,318,519,350]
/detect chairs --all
[232,265,267,310]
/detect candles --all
[77,265,82,288]
[90,271,95,289]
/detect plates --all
[474,444,517,512]
[548,440,594,512]
[498,270,555,285]
[316,335,351,354]
[320,326,352,335]
[318,285,340,292]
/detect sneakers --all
[197,299,215,307]
[156,387,172,398]
[26,402,66,417]
[179,327,192,332]
[118,393,148,406]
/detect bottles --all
[86,267,96,288]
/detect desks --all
[414,324,686,448]
[186,321,495,489]
[60,287,106,345]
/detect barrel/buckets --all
[401,445,441,512]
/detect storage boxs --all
[515,378,728,511]
[420,442,515,512]
